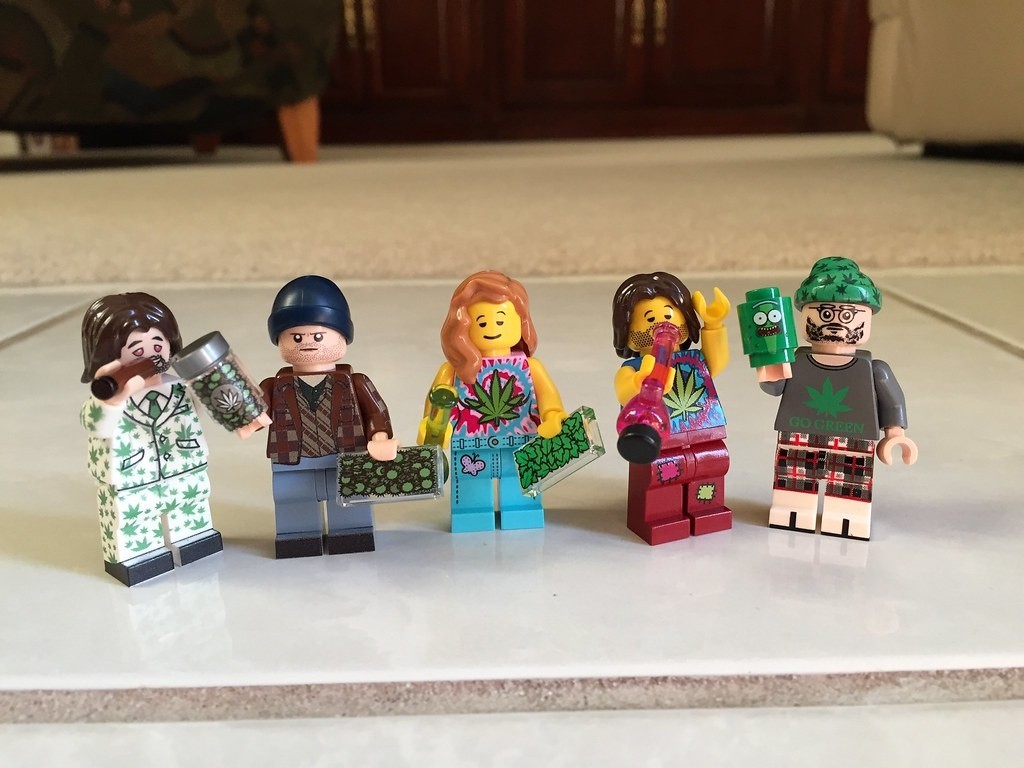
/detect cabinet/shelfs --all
[322,0,874,141]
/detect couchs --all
[0,0,341,161]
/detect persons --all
[80,293,222,587]
[417,271,569,533]
[233,274,398,559]
[756,258,917,540]
[613,271,733,548]
